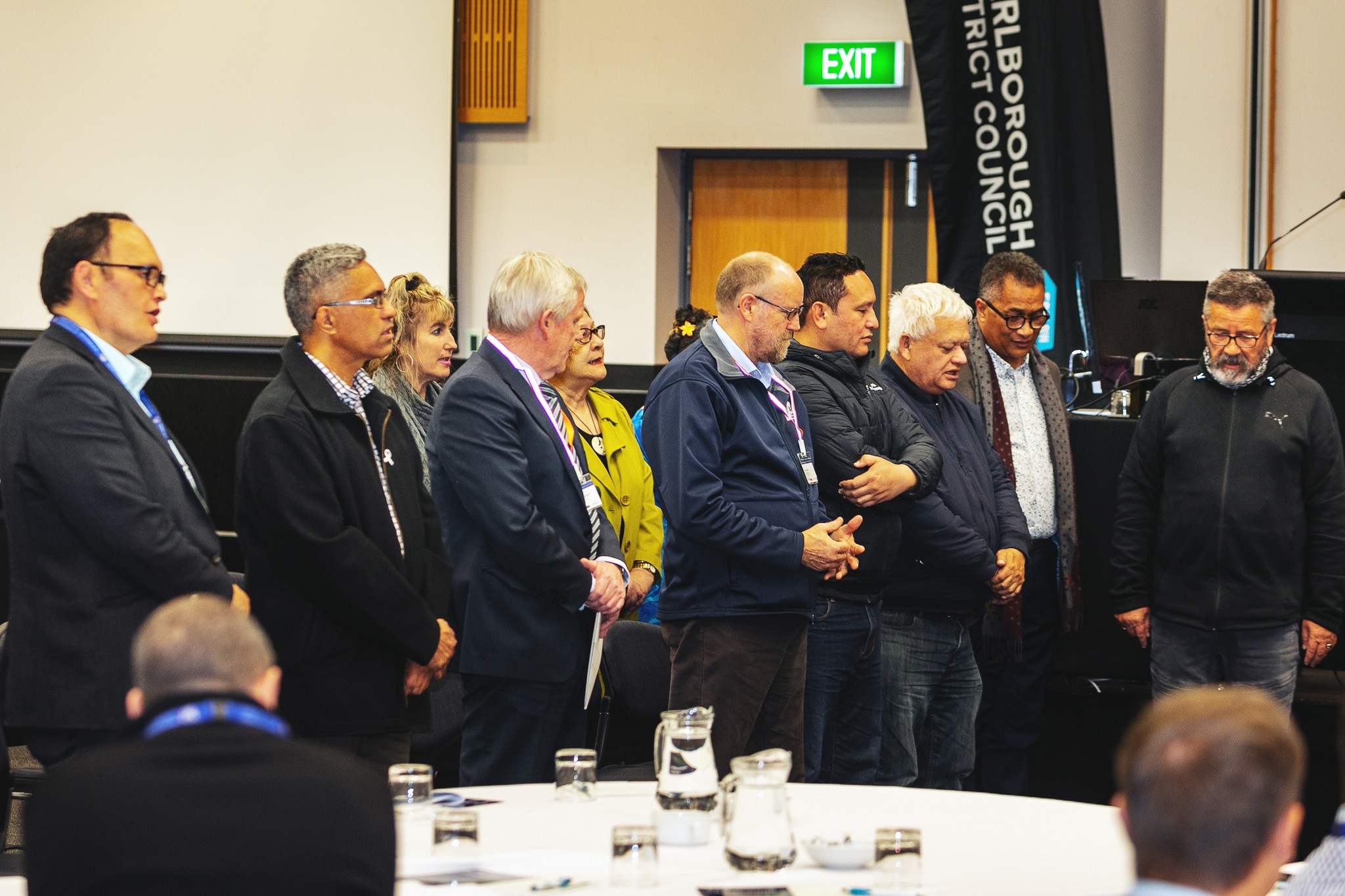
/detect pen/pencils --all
[532,879,570,891]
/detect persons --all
[0,211,1344,896]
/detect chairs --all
[604,619,672,779]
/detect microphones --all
[1259,191,1345,269]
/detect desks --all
[394,779,1137,896]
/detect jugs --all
[654,705,719,811]
[719,748,797,872]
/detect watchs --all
[633,562,656,577]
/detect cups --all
[653,810,710,845]
[610,824,659,887]
[431,811,479,868]
[873,826,925,890]
[554,749,596,802]
[1113,390,1130,416]
[389,763,432,820]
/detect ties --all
[540,381,602,561]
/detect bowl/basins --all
[802,836,876,870]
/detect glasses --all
[980,297,1050,331]
[578,325,606,344]
[737,294,804,321]
[311,289,385,321]
[65,261,166,287]
[1206,318,1271,348]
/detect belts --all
[881,607,962,622]
